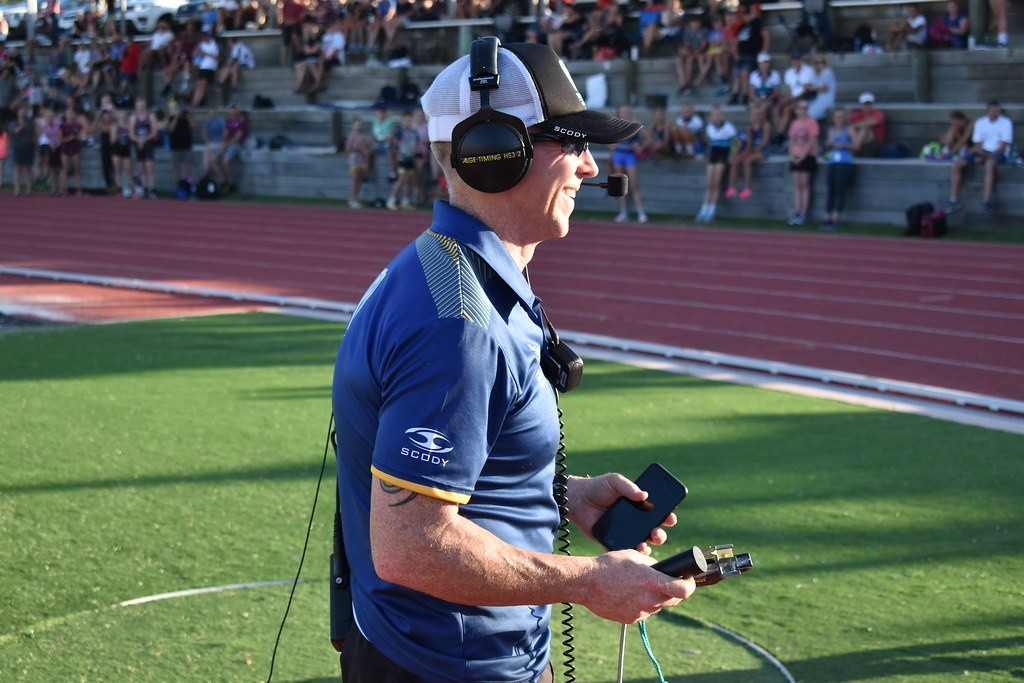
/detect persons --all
[208,37,255,93]
[333,42,698,683]
[0,0,1024,228]
[850,92,886,157]
[671,103,704,159]
[884,5,927,53]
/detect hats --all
[757,53,770,63]
[858,91,874,104]
[421,42,643,145]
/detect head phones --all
[449,35,535,194]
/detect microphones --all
[582,173,629,197]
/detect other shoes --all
[819,221,839,232]
[980,200,991,211]
[401,200,416,210]
[942,201,963,215]
[385,198,398,211]
[14,180,158,201]
[694,213,714,225]
[614,215,629,222]
[724,187,737,198]
[638,215,649,224]
[739,188,752,198]
[678,81,748,110]
[789,213,806,225]
[349,200,362,209]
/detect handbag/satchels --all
[905,203,947,239]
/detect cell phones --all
[592,462,688,551]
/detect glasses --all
[529,133,588,155]
